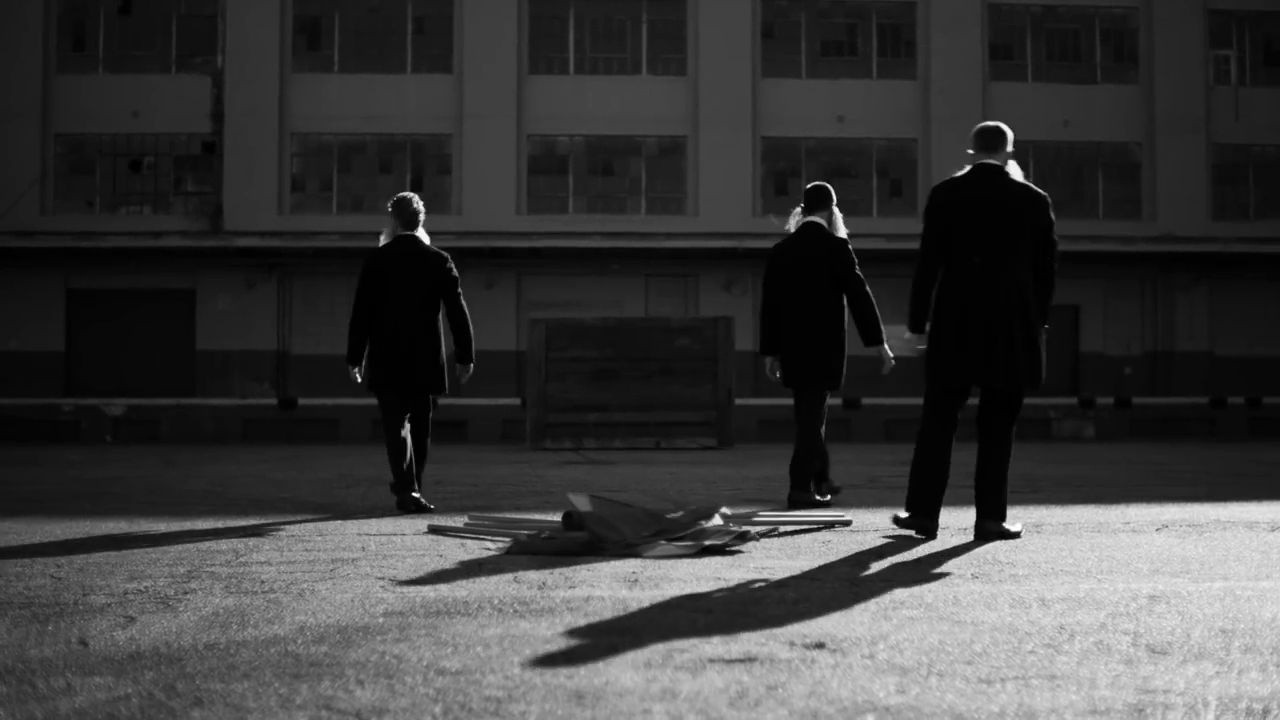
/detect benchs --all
[525,314,736,452]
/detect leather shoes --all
[893,514,938,538]
[974,519,1024,543]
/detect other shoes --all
[789,494,833,508]
[395,492,436,513]
[815,478,841,497]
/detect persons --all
[759,180,897,509]
[890,120,1061,540]
[346,191,478,515]
